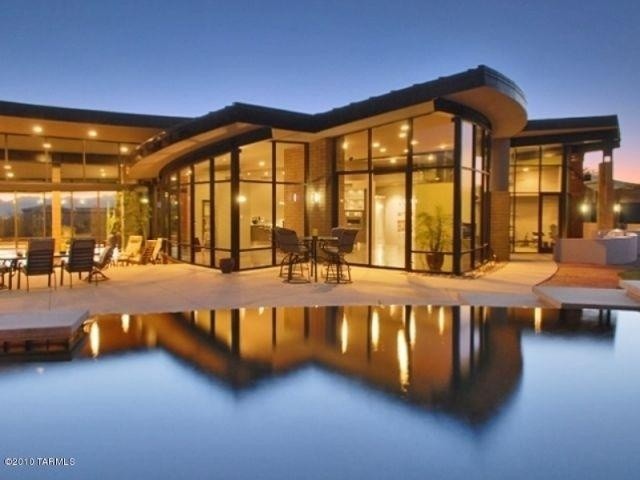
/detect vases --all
[220,258,235,273]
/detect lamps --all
[582,167,591,181]
[374,186,386,200]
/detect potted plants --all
[413,204,454,273]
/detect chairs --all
[193,238,205,261]
[247,224,361,285]
[519,233,529,248]
[0,233,165,293]
[202,239,211,249]
